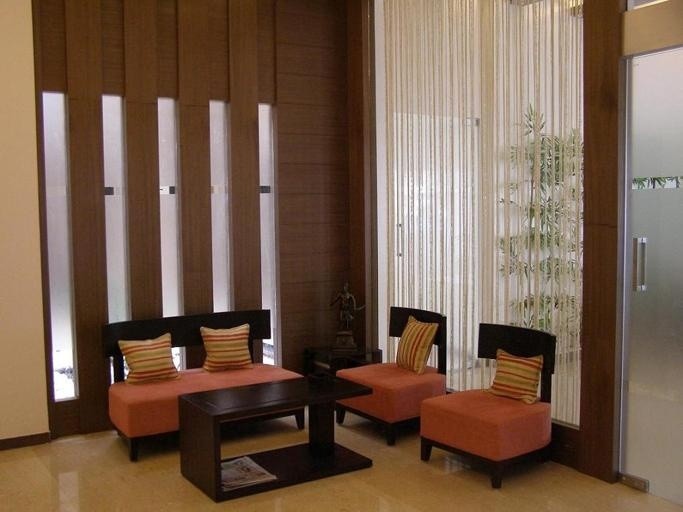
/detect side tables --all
[300,347,369,383]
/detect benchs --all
[98,310,308,463]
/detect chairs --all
[336,306,447,447]
[419,322,556,489]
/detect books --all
[220,456,277,492]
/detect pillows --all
[491,349,544,405]
[397,314,438,374]
[200,323,255,371]
[115,332,181,383]
